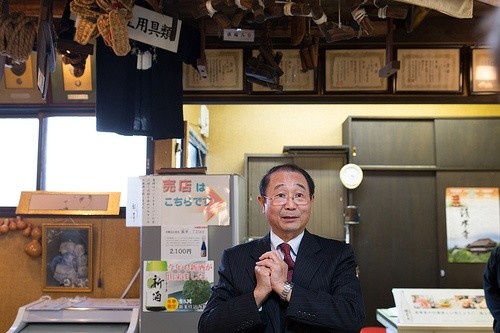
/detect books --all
[375,288,494,333]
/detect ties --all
[277,243,295,284]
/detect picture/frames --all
[41,224,95,293]
[181,42,500,104]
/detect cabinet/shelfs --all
[153,121,207,173]
[245,116,500,327]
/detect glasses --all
[263,194,311,206]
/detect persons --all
[483,244,500,333]
[198,163,363,333]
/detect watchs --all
[280,280,295,301]
